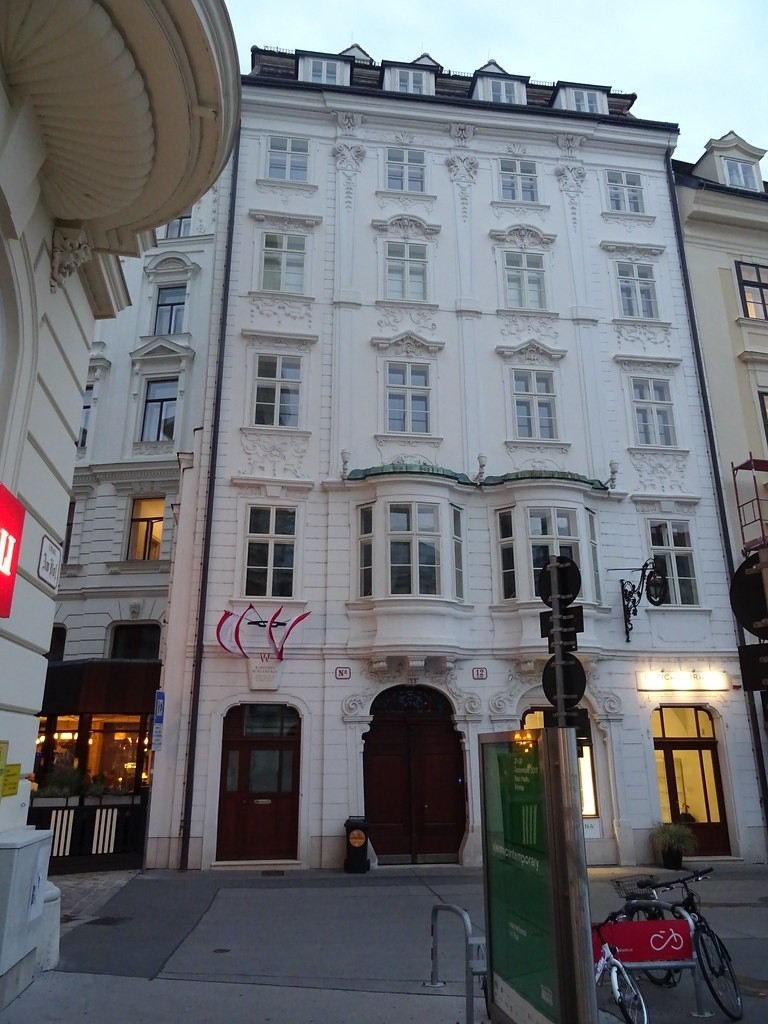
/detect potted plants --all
[649,822,699,870]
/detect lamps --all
[474,451,487,483]
[340,449,350,479]
[620,559,667,643]
[604,458,619,489]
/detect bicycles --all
[610,866,743,1020]
[589,906,649,1024]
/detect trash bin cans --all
[344,815,371,874]
